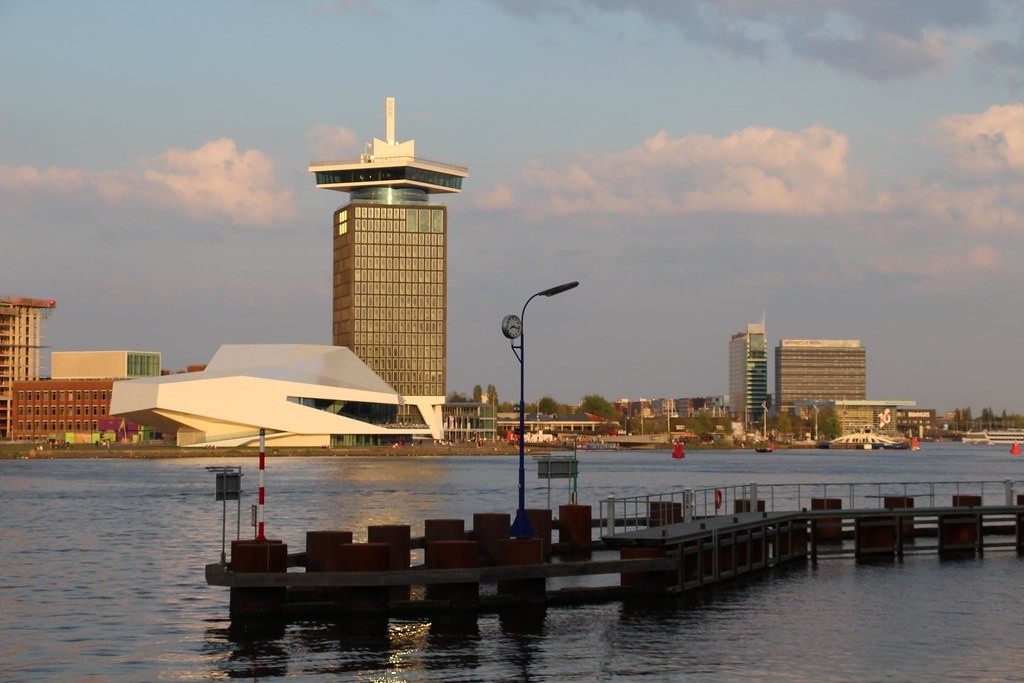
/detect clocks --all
[502,314,522,339]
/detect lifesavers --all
[715,489,722,507]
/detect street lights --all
[762,400,768,443]
[500,282,579,520]
[813,403,820,440]
